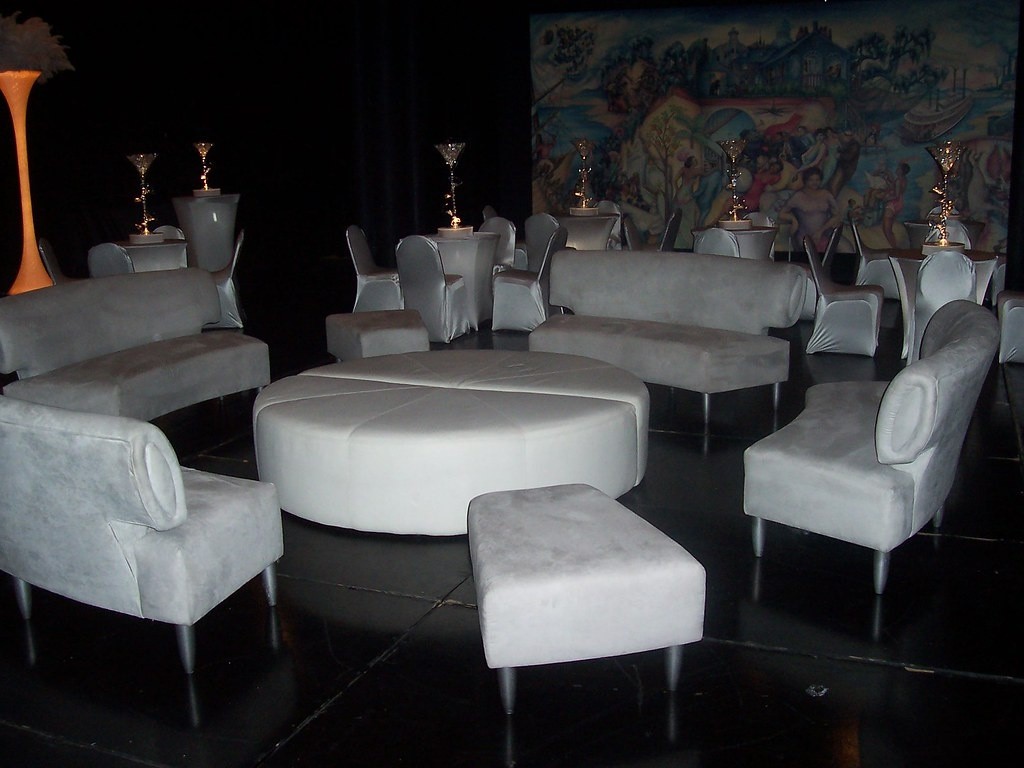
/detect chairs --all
[32,199,1022,366]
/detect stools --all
[323,310,431,367]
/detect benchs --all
[467,485,703,721]
[2,269,272,419]
[748,293,997,597]
[534,252,809,424]
[0,402,283,736]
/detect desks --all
[255,348,650,538]
[904,219,985,251]
[114,240,188,271]
[888,254,996,360]
[691,225,779,263]
[425,234,499,331]
